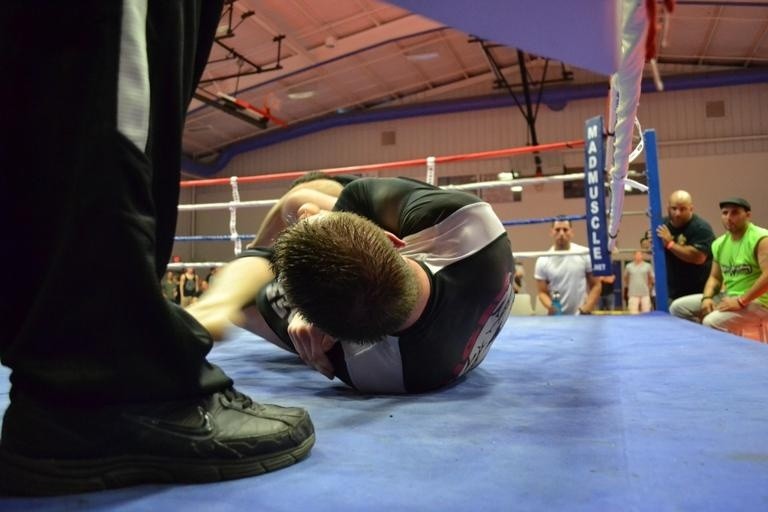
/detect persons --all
[0,0,315,498]
[656,190,716,306]
[184,171,516,395]
[624,229,655,312]
[669,197,768,332]
[533,215,603,315]
[161,267,216,308]
[598,271,616,311]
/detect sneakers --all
[0,385,317,502]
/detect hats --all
[719,197,751,211]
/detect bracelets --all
[737,297,746,308]
[701,296,712,302]
[666,240,675,249]
